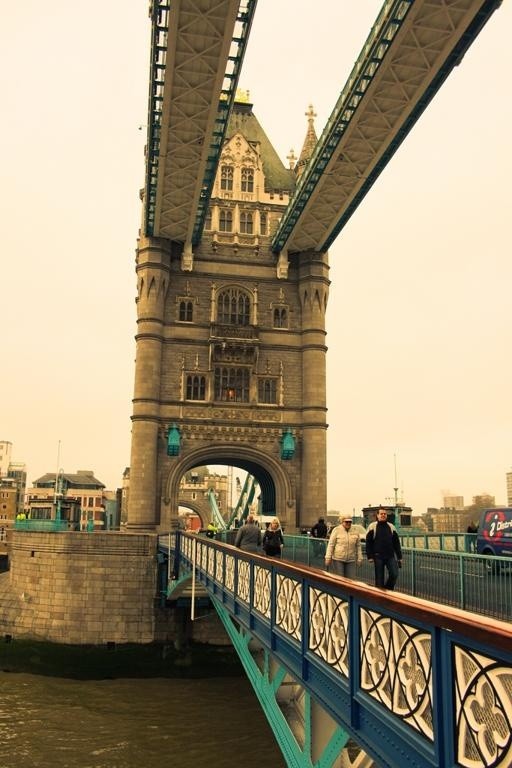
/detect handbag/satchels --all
[206,530,213,538]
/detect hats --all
[342,514,353,522]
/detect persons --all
[363,506,403,589]
[322,514,364,580]
[311,517,327,540]
[16,511,32,521]
[261,518,284,559]
[466,519,477,553]
[234,514,262,558]
[206,522,220,538]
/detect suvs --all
[476,508,512,572]
